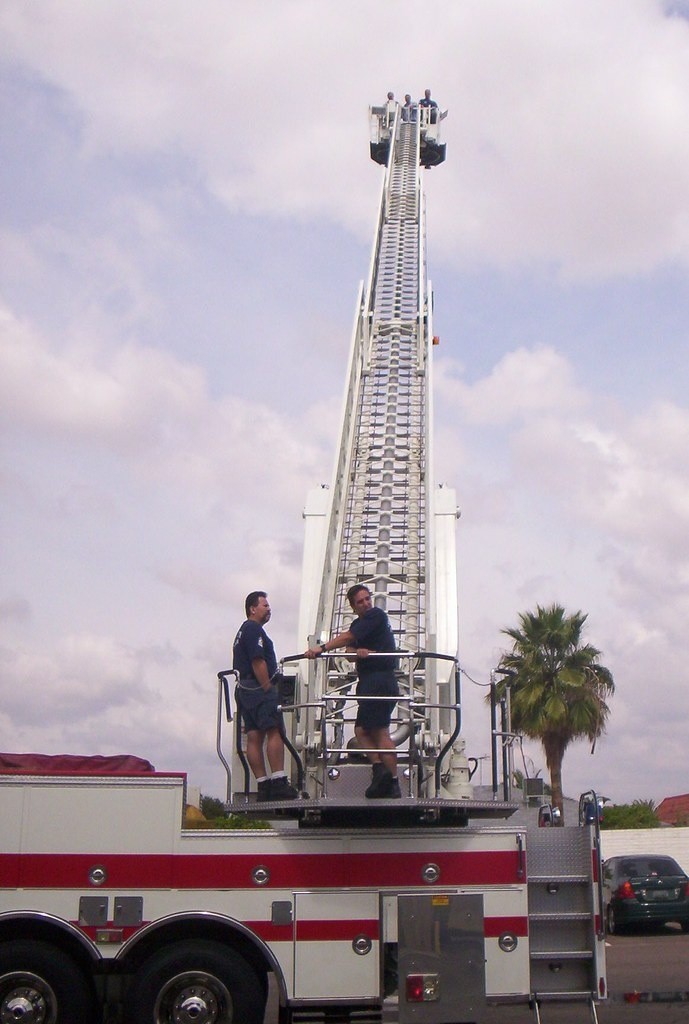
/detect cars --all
[600,855,688,938]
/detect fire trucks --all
[0,105,607,1024]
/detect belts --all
[239,673,256,680]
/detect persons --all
[305,585,400,799]
[232,591,298,802]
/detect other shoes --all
[257,776,298,802]
[366,762,401,799]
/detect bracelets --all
[320,643,327,652]
